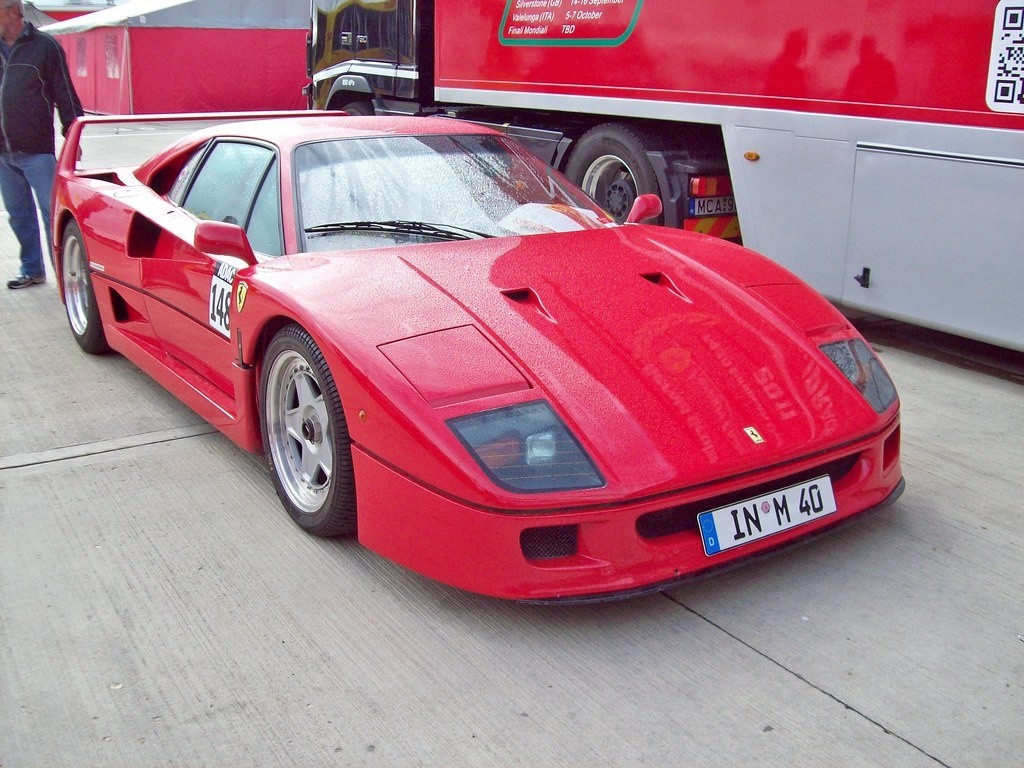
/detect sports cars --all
[49,107,907,611]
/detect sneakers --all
[8,274,46,289]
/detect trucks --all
[297,2,1024,362]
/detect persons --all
[0,0,84,288]
